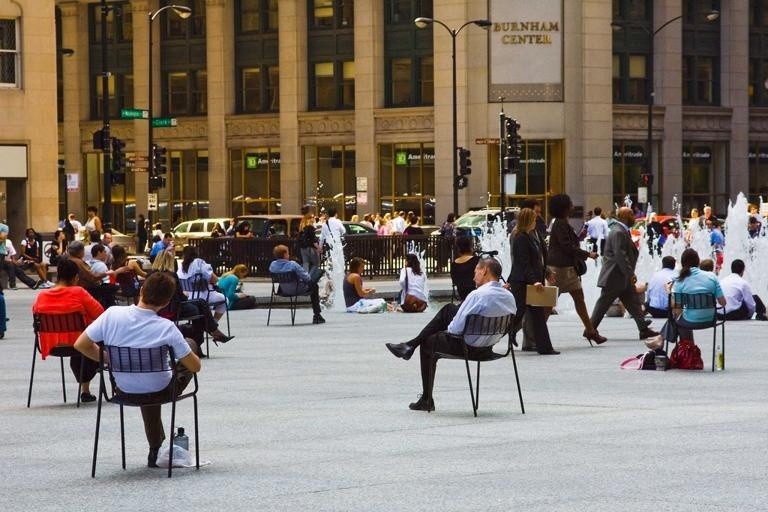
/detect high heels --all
[585,330,608,348]
[213,335,236,347]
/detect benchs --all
[0,264,61,289]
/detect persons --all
[269,204,456,324]
[501,195,768,355]
[0,207,256,403]
[386,258,516,410]
[450,237,481,301]
[75,272,203,469]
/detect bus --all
[123,201,211,233]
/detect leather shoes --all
[148,446,161,468]
[640,328,661,340]
[409,394,435,411]
[385,342,415,361]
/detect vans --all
[171,216,232,238]
[235,214,306,236]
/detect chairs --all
[265,271,318,328]
[80,271,231,363]
[27,312,106,411]
[663,292,727,373]
[426,311,527,422]
[88,339,200,479]
[450,280,475,308]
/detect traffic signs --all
[147,192,157,211]
[150,118,177,128]
[120,109,149,120]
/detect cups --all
[653,356,665,371]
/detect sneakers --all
[11,287,18,290]
[512,333,518,347]
[39,280,56,289]
[522,346,538,351]
[32,279,42,290]
[80,391,97,402]
[539,350,561,355]
[313,314,325,324]
[644,339,664,352]
[755,314,768,321]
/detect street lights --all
[414,17,491,220]
[611,9,720,212]
[145,5,193,249]
[56,47,75,60]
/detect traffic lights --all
[111,137,126,169]
[153,146,168,173]
[459,148,472,177]
[111,172,123,185]
[506,120,522,156]
[504,156,520,172]
[151,175,163,190]
[456,177,468,189]
[641,174,654,185]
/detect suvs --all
[428,204,522,263]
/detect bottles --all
[714,343,723,372]
[172,427,190,453]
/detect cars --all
[629,215,691,251]
[315,220,386,261]
[233,190,430,212]
